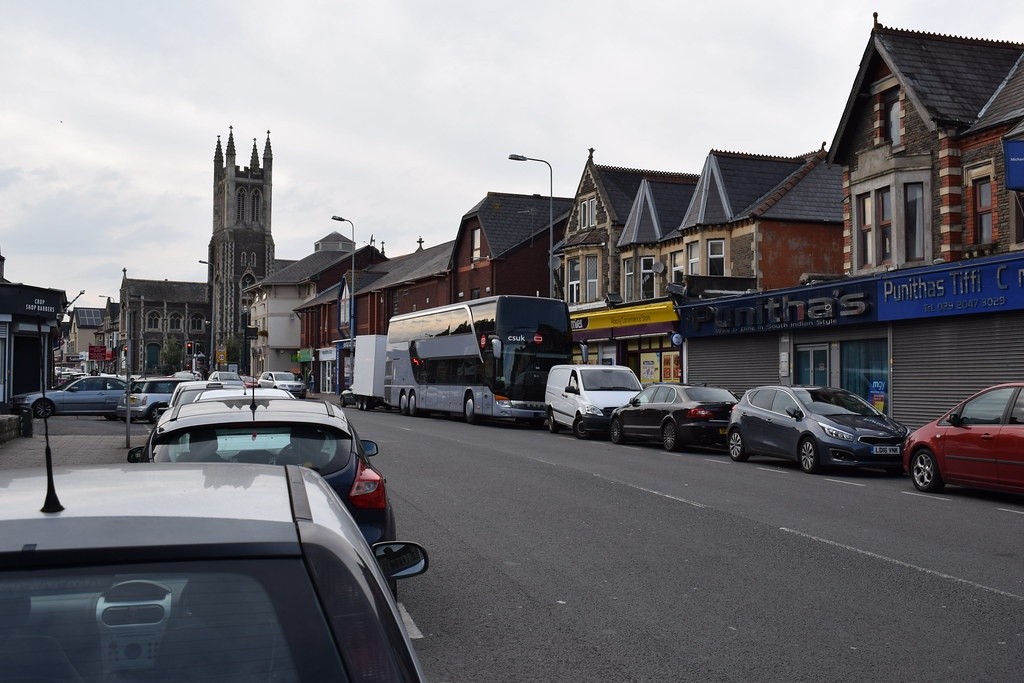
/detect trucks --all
[352,335,388,411]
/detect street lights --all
[508,153,553,298]
[331,215,353,388]
[198,260,214,376]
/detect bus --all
[384,295,590,425]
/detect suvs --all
[116,378,195,425]
[207,370,246,390]
[257,372,306,399]
[127,398,398,604]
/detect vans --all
[543,365,648,440]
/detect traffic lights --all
[186,341,194,356]
[123,345,128,357]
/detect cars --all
[177,387,296,456]
[54,365,143,382]
[240,375,259,389]
[1,461,431,683]
[900,382,1024,494]
[609,384,741,455]
[169,381,247,408]
[9,375,143,421]
[339,383,356,407]
[167,370,202,381]
[726,386,911,477]
